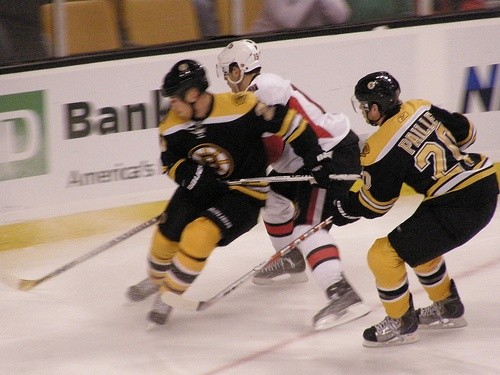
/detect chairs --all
[39,0,488,58]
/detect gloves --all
[304,146,336,190]
[178,159,230,200]
[327,193,363,226]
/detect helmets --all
[161,59,205,97]
[353,71,401,114]
[217,39,261,75]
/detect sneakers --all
[363,291,419,347]
[251,247,309,285]
[412,278,469,328]
[144,291,176,333]
[124,276,162,302]
[311,271,371,331]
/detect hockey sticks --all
[160,215,336,312]
[0,213,163,292]
[218,172,361,186]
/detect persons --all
[126,59,333,325]
[216,39,370,331]
[329,70,500,348]
[253,0,349,33]
